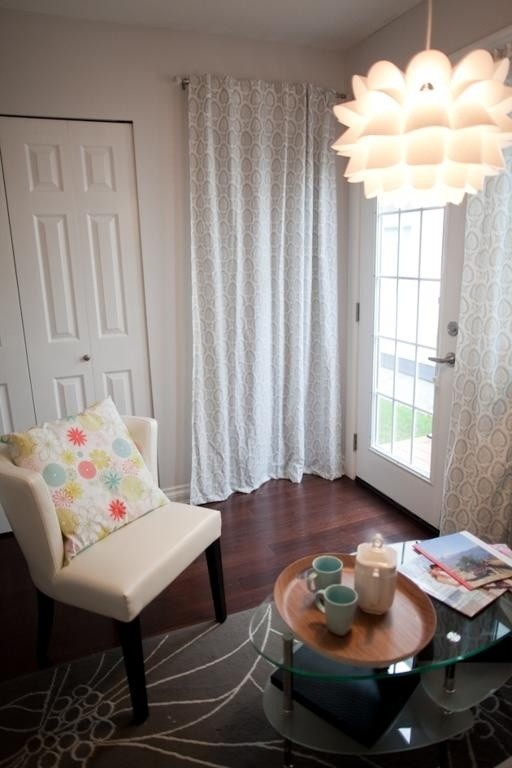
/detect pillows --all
[0,396,169,568]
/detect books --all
[396,528,511,619]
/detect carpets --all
[0,601,512,768]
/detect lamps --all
[330,0,512,206]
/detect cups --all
[306,556,356,637]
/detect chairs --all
[0,413,227,724]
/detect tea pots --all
[355,535,399,615]
